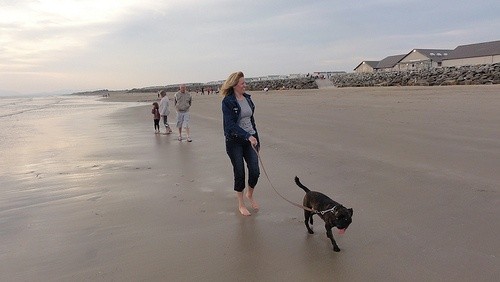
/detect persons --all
[152,102,160,133]
[159,90,172,134]
[189,86,220,95]
[221,71,260,216]
[174,85,192,142]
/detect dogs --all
[294,175,354,252]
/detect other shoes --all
[187,137,192,142]
[178,136,182,141]
